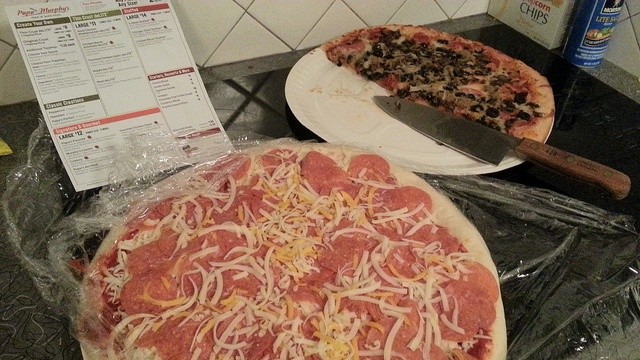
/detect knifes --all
[372,95,631,199]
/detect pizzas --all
[320,23,556,144]
[79,137,507,360]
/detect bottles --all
[560,0,625,68]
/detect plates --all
[285,45,557,176]
